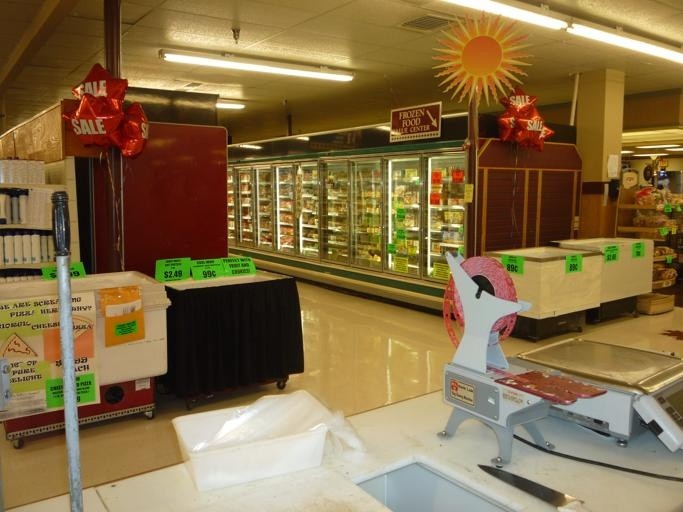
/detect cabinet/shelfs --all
[614,181,683,290]
[0,155,80,269]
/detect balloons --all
[60,62,149,159]
[495,84,555,153]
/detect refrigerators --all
[480,237,653,320]
[1,271,168,449]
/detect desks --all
[159,272,304,403]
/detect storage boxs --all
[636,293,676,315]
[171,405,327,492]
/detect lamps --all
[158,48,354,82]
[441,0,683,73]
[215,99,245,110]
